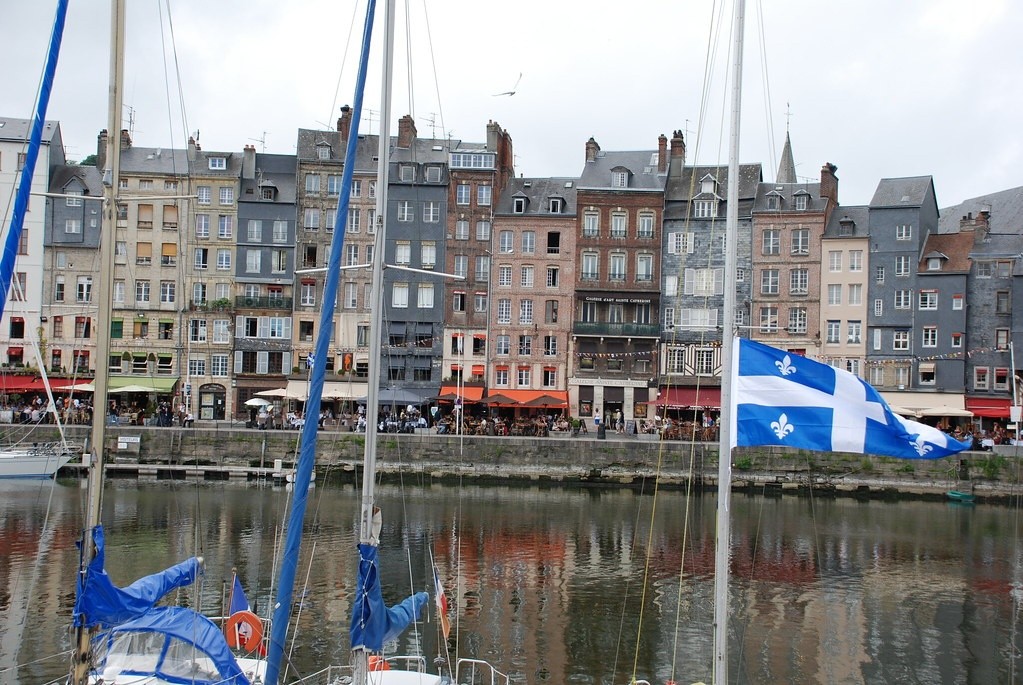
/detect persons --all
[0,394,194,428]
[594,407,625,434]
[640,411,720,441]
[286,404,427,434]
[936,420,1011,452]
[433,407,572,437]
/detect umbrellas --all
[477,393,519,417]
[523,394,567,415]
[244,388,299,405]
[108,385,164,394]
[430,392,473,417]
[357,386,429,406]
[52,383,95,393]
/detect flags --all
[229,579,267,658]
[430,546,450,638]
[731,340,974,461]
[308,353,315,365]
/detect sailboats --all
[0,0,82,481]
[50,0,512,685]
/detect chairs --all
[659,426,716,441]
[255,415,291,429]
[434,420,544,436]
[13,409,138,425]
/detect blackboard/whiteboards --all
[581,418,588,434]
[626,419,636,434]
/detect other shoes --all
[615,432,621,435]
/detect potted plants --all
[639,419,645,431]
[212,298,229,313]
[349,368,356,379]
[122,351,132,374]
[572,420,580,433]
[146,353,156,372]
[338,368,345,379]
[292,367,300,375]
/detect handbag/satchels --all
[176,411,183,417]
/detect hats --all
[59,396,62,400]
[615,409,620,412]
[184,413,188,417]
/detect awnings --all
[285,381,369,400]
[659,386,721,410]
[488,389,568,408]
[439,387,484,404]
[0,375,180,392]
[879,406,974,417]
[970,407,1011,417]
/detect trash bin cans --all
[597,422,607,439]
